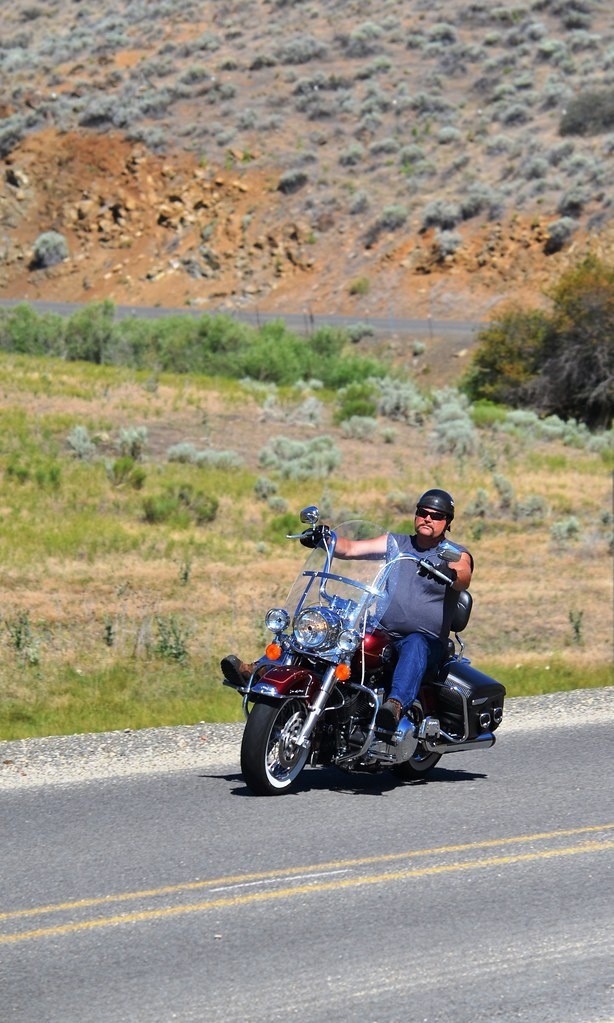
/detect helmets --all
[417,489,455,520]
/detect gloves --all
[300,525,329,549]
[427,560,457,585]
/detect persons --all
[220,489,475,731]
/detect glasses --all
[415,508,446,520]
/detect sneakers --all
[221,655,267,702]
[374,699,402,740]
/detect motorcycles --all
[223,506,507,796]
